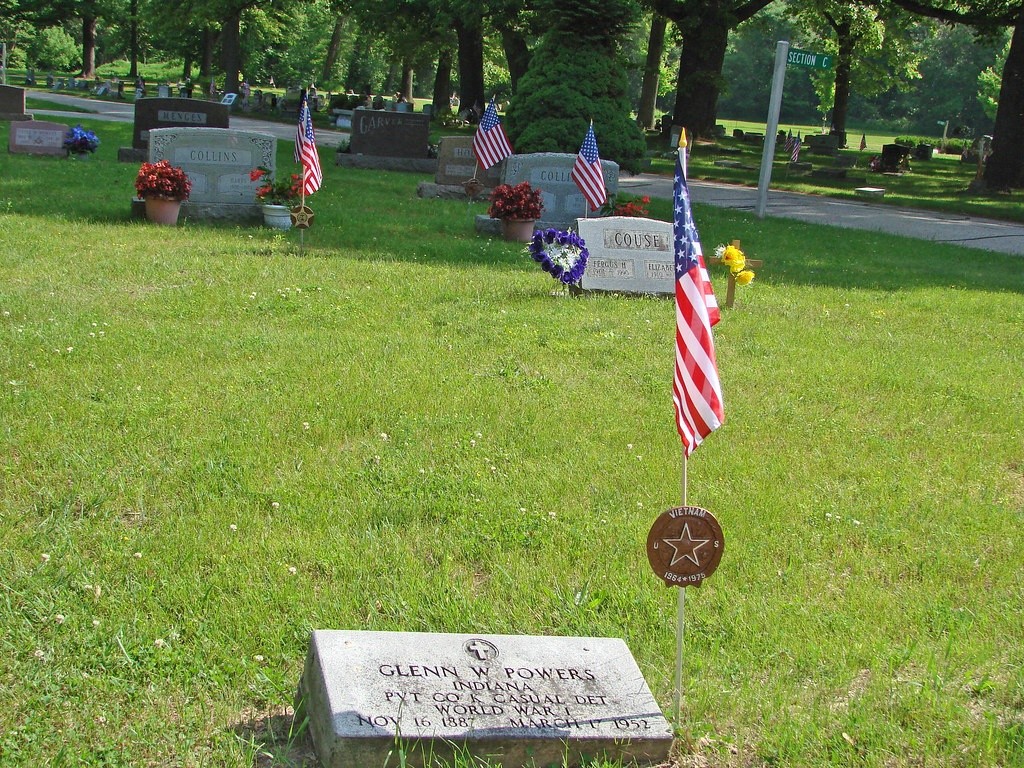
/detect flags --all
[295,93,322,196]
[783,129,794,152]
[571,125,608,212]
[472,98,515,171]
[790,131,802,162]
[673,149,725,459]
[859,133,867,151]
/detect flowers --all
[63,124,99,152]
[531,228,589,282]
[869,155,881,172]
[136,160,191,200]
[898,155,914,173]
[713,240,754,287]
[603,188,650,218]
[487,181,546,220]
[249,162,309,206]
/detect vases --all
[145,194,181,225]
[69,150,90,161]
[263,205,291,227]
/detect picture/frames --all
[500,218,535,243]
